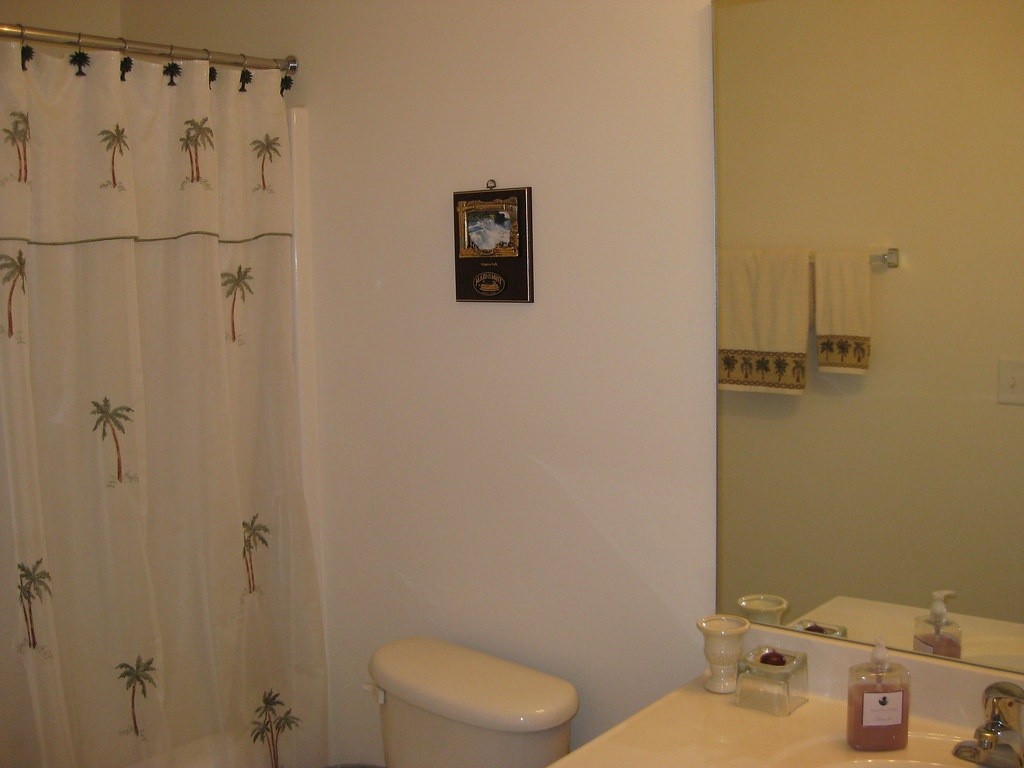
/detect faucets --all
[973,682,1024,759]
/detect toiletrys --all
[846,635,912,751]
[910,587,963,659]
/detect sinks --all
[949,623,1024,678]
[770,727,972,768]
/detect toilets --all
[367,630,579,768]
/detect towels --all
[813,250,874,377]
[717,242,812,397]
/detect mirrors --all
[708,2,1023,680]
